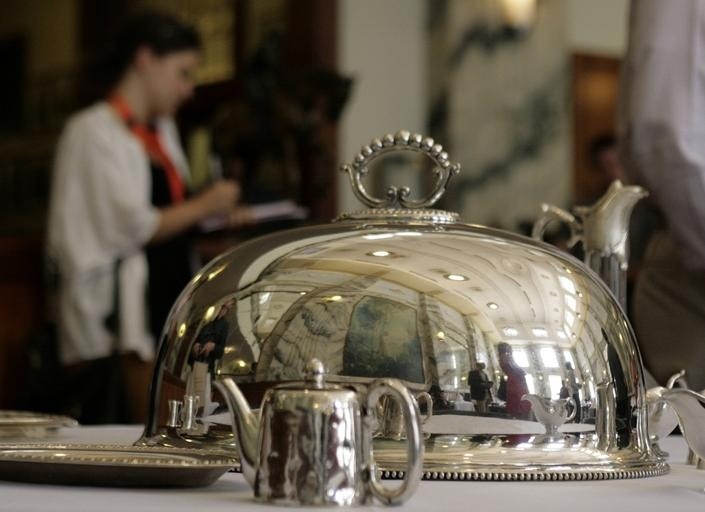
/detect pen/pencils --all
[212,161,221,180]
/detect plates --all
[0,442,237,488]
[0,410,80,439]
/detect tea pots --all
[213,358,424,510]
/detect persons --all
[560,380,569,398]
[43,15,242,424]
[626,0,705,404]
[498,343,530,419]
[601,328,630,450]
[145,319,177,437]
[565,362,581,423]
[580,132,647,281]
[468,363,493,413]
[187,298,238,380]
[428,357,439,397]
